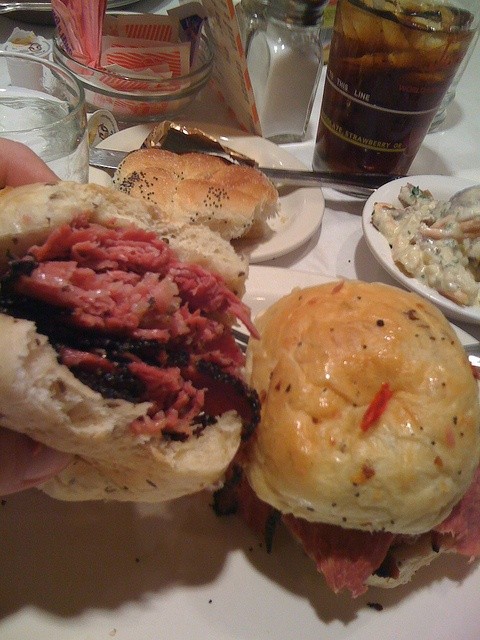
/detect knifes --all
[90,146,408,195]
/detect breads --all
[117,148,279,242]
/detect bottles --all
[244,0,324,144]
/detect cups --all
[0,52,89,185]
[312,0,479,196]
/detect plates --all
[90,118,325,264]
[363,175,480,324]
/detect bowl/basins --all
[59,11,214,115]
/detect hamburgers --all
[243,277,480,594]
[1,181,263,505]
